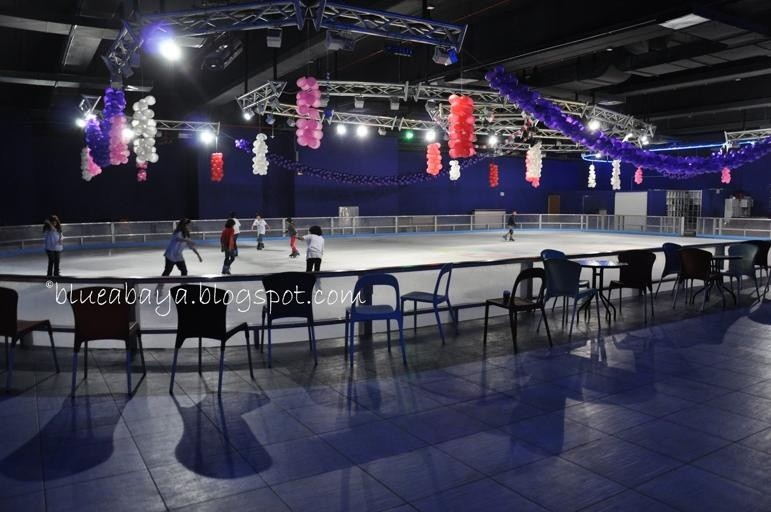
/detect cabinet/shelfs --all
[472,209,506,229]
[724,199,751,219]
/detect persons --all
[155,219,193,294]
[252,216,270,250]
[231,213,241,256]
[300,225,324,290]
[220,220,236,273]
[503,211,517,241]
[286,218,299,256]
[254,210,262,215]
[44,216,64,276]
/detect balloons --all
[81,89,158,183]
[234,67,771,190]
[210,152,224,183]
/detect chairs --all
[169,284,254,400]
[67,286,146,399]
[260,271,318,368]
[343,238,771,367]
[0,285,60,392]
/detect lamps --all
[243,28,458,136]
